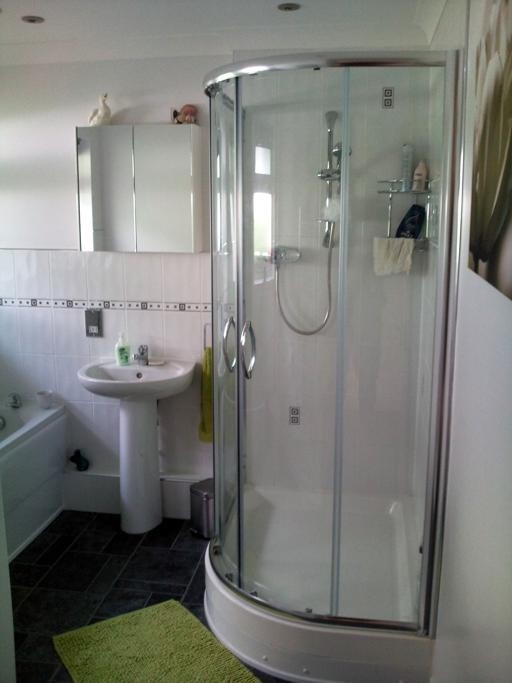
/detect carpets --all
[50,599,261,683]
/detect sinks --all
[78,353,197,399]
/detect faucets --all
[4,392,22,408]
[131,344,149,365]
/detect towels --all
[197,347,213,444]
[373,236,415,276]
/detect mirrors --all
[74,123,203,255]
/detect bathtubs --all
[0,400,75,562]
